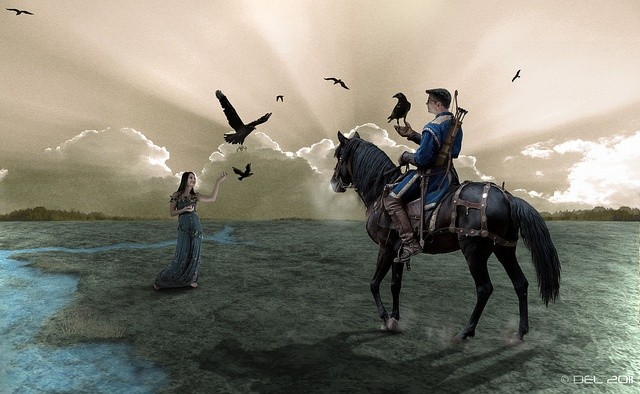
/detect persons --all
[154,171,227,291]
[382,88,462,263]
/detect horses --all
[330,130,561,343]
